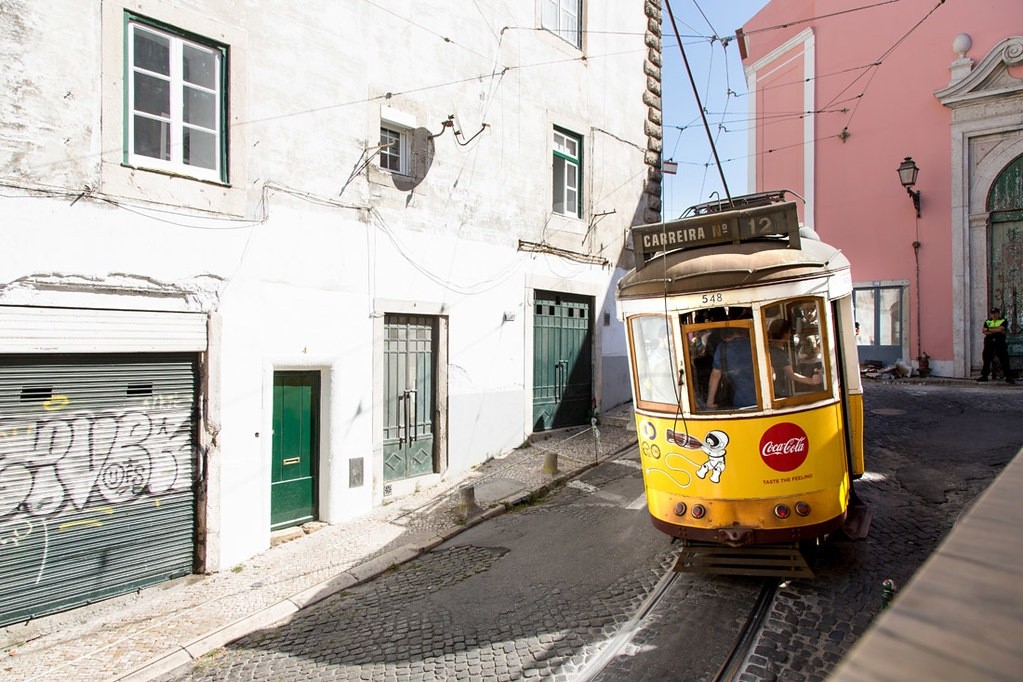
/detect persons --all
[707,327,757,408]
[690,307,727,397]
[977,308,1015,385]
[767,319,820,399]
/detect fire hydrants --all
[916,351,932,378]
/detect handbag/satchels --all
[714,342,734,407]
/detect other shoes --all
[976,376,988,381]
[1006,377,1015,383]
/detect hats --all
[990,308,999,313]
[703,307,726,316]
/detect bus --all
[614,0,864,582]
